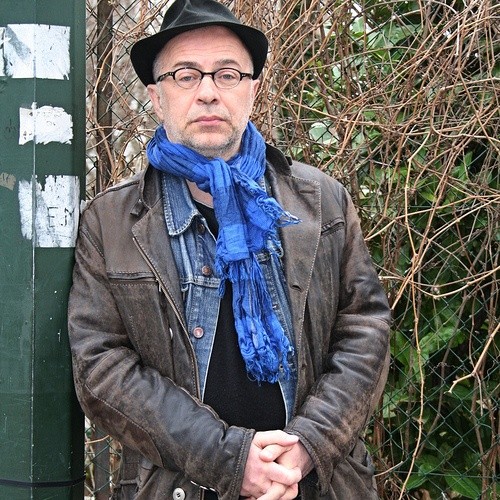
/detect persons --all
[68,0,392,500]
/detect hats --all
[130,0,268,87]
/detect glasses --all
[158,67,252,89]
